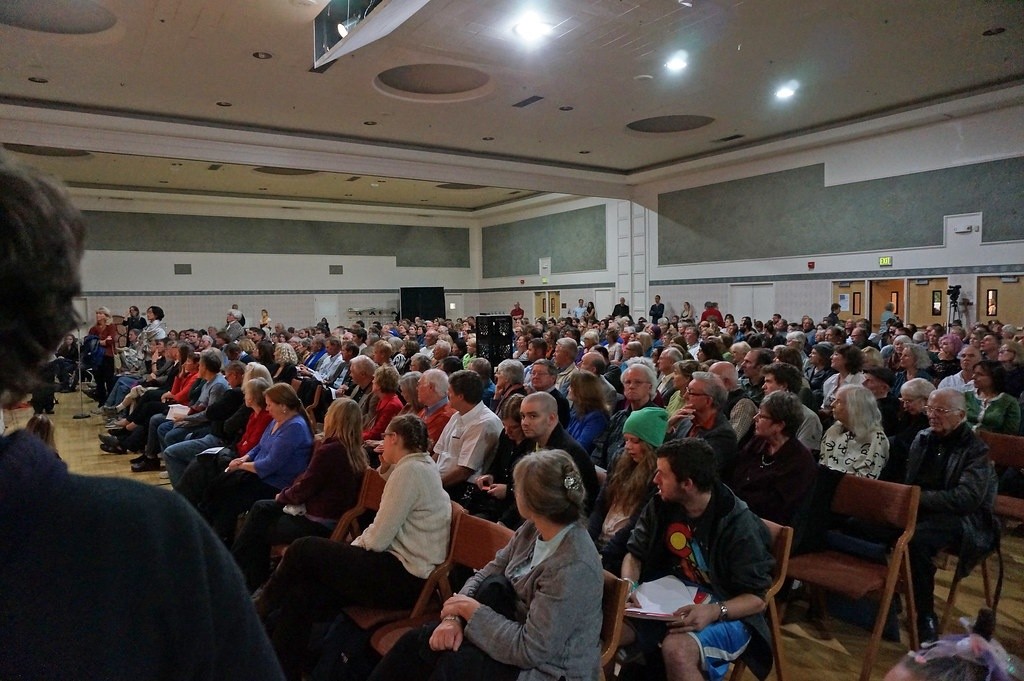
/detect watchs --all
[716,601,729,622]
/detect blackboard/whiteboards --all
[400,286,446,321]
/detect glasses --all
[530,370,555,377]
[901,395,926,403]
[926,405,966,417]
[380,433,395,440]
[685,386,715,400]
[757,409,787,426]
[622,378,653,387]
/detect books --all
[624,593,711,622]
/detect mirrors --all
[891,291,898,314]
[932,290,942,316]
[986,288,997,316]
[852,292,861,315]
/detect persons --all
[29,295,1024,681]
[0,167,286,681]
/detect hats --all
[622,407,669,448]
[880,345,895,359]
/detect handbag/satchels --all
[416,573,521,681]
[111,342,122,370]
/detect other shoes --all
[101,406,117,416]
[34,409,44,414]
[131,458,161,472]
[918,613,939,651]
[98,433,120,448]
[114,418,138,430]
[44,409,54,414]
[159,470,169,479]
[130,453,159,464]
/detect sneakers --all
[100,443,128,454]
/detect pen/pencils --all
[625,583,638,602]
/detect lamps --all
[337,17,359,38]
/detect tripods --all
[948,300,960,332]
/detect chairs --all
[275,432,1024,681]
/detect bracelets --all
[441,614,462,622]
